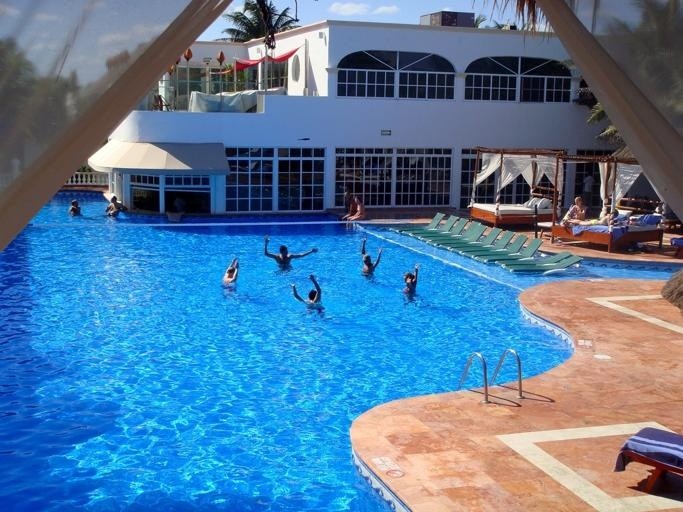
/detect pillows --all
[522,197,540,208]
[638,214,661,226]
[616,208,632,218]
[532,198,552,209]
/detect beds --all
[465,144,565,228]
[550,152,665,253]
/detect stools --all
[669,237,683,259]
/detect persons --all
[221,256,239,288]
[288,273,322,305]
[400,263,420,295]
[564,209,623,227]
[561,195,588,223]
[345,195,366,230]
[335,192,356,228]
[165,205,186,224]
[359,234,383,278]
[104,195,128,218]
[67,199,81,217]
[263,235,319,267]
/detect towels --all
[571,224,628,240]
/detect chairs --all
[536,204,588,240]
[388,211,584,275]
[616,424,682,495]
